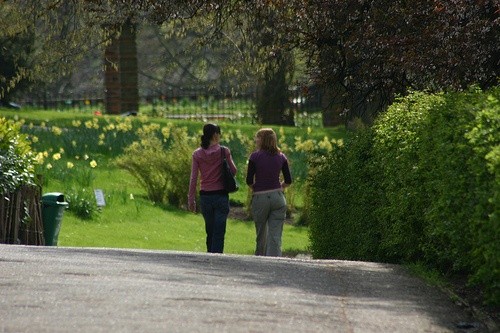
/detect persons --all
[247,128,292,257]
[188,123,237,253]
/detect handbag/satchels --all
[220,146,239,193]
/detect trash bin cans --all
[40,190,70,246]
